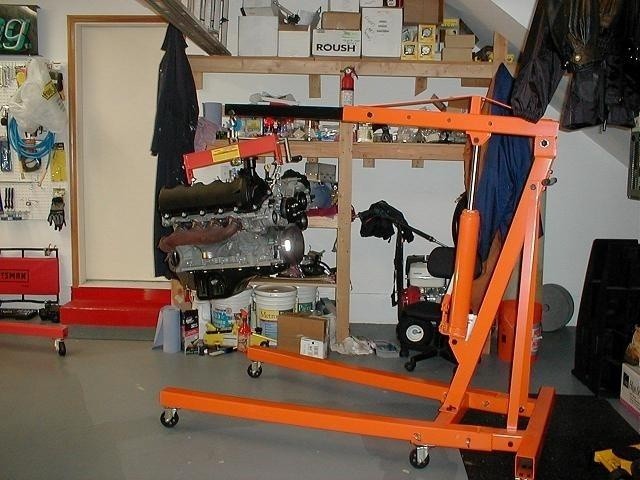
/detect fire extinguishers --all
[340,67,358,107]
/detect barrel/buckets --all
[294,286,320,313]
[498,301,543,364]
[253,284,298,348]
[211,288,252,346]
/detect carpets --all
[453,394,640,480]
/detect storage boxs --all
[328,0,359,13]
[278,24,311,57]
[362,8,402,57]
[403,0,439,25]
[243,0,279,16]
[238,16,279,56]
[444,36,475,48]
[322,11,360,30]
[442,48,472,62]
[313,29,361,57]
[618,363,640,419]
[276,313,329,360]
[360,0,382,7]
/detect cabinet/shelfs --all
[183,56,525,343]
